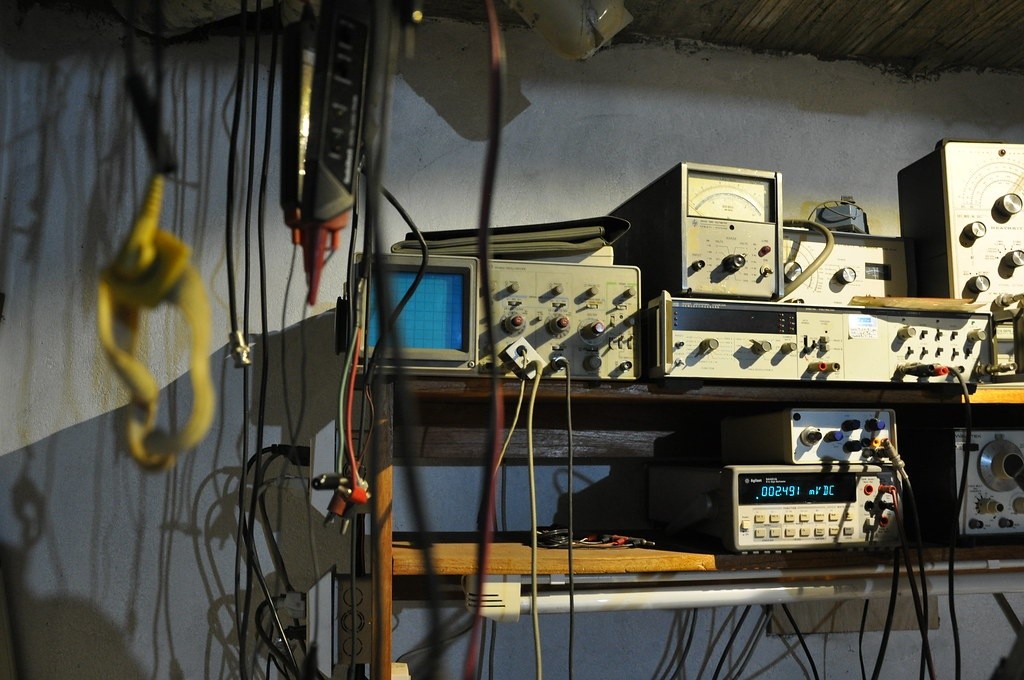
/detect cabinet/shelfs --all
[336,366,1024,680]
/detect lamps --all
[464,559,1024,626]
[507,0,635,62]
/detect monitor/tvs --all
[358,253,478,368]
